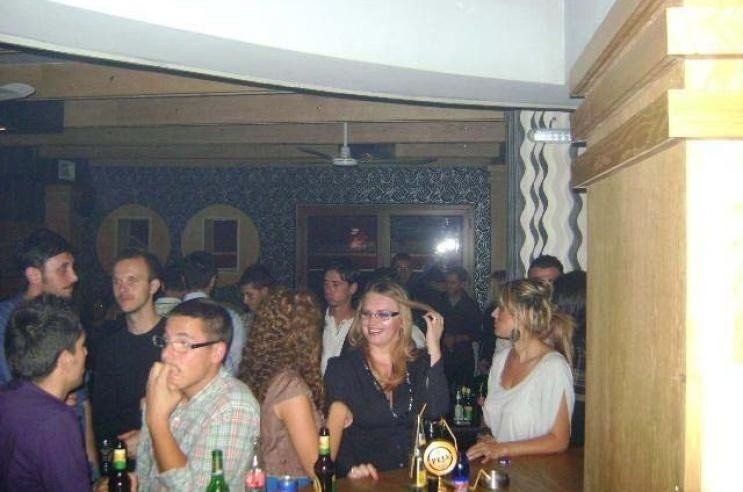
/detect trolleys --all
[291,120,437,167]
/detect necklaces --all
[360,357,412,416]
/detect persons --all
[237,290,354,492]
[323,280,451,481]
[464,278,575,465]
[0,227,586,492]
[135,296,259,492]
[3,296,100,492]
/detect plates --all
[294,202,476,300]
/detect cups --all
[152,334,219,352]
[359,310,399,320]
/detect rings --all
[351,468,353,473]
[433,318,439,321]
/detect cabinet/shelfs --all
[241,441,269,491]
[276,454,299,492]
[205,448,230,492]
[406,383,480,492]
[310,416,338,491]
[95,431,137,491]
